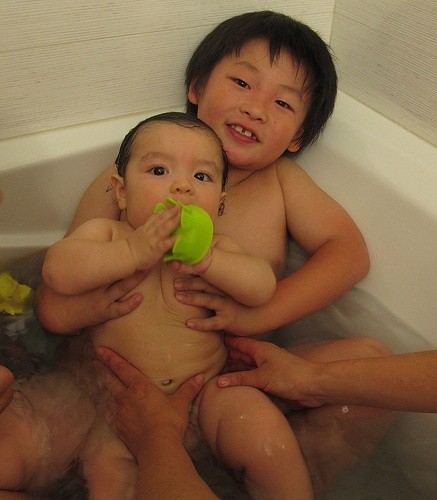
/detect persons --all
[35,111,317,500]
[75,334,437,500]
[0,10,371,497]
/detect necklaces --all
[217,170,257,216]
